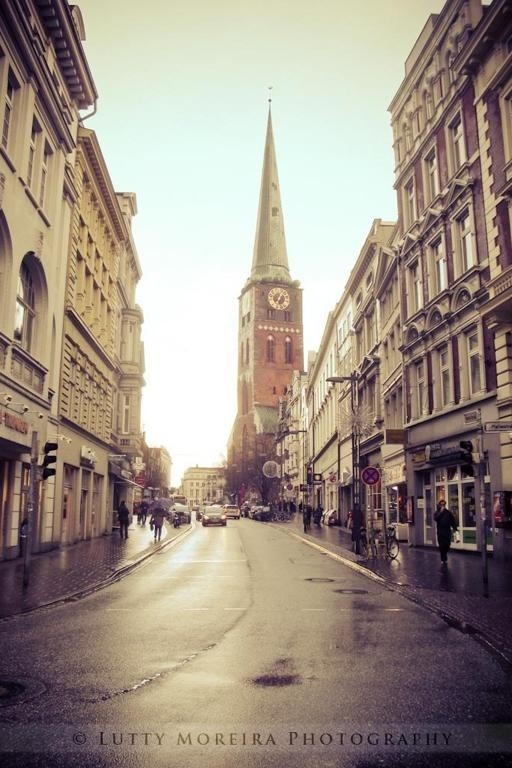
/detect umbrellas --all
[150,498,175,508]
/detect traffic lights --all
[37,441,58,481]
[458,438,479,479]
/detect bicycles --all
[271,510,290,523]
[353,525,400,559]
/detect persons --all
[173,515,180,528]
[306,501,313,529]
[149,507,170,540]
[143,500,149,524]
[432,499,460,564]
[136,500,145,522]
[351,502,365,555]
[117,499,130,539]
[313,503,325,526]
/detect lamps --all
[3,396,95,455]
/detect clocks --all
[268,287,291,310]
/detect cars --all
[132,496,241,527]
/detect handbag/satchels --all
[150,524,154,531]
